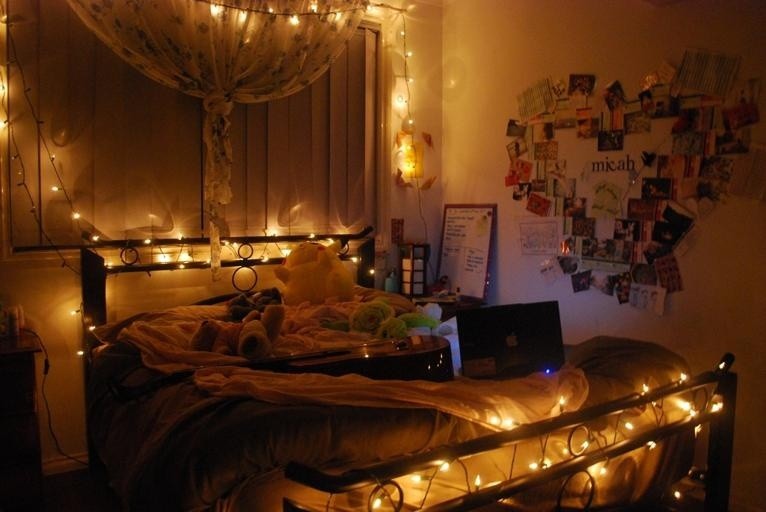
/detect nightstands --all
[378,287,492,321]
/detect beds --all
[81,237,736,512]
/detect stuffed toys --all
[319,296,408,334]
[272,236,355,311]
[190,301,284,361]
[377,311,453,338]
[426,273,456,300]
[227,283,281,321]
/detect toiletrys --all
[17,306,26,333]
[7,306,19,337]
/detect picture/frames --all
[436,204,494,301]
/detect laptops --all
[454,300,567,382]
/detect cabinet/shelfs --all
[0,329,44,512]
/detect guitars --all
[96,333,452,407]
